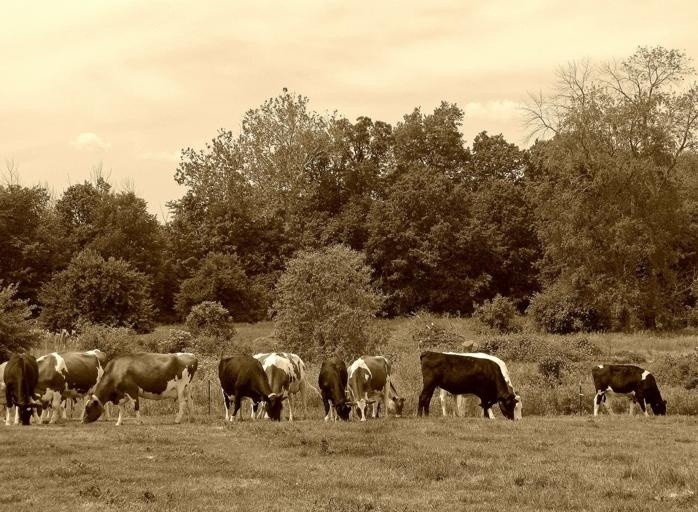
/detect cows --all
[591,364,668,417]
[218,351,407,423]
[417,351,519,422]
[0,349,107,426]
[80,352,200,426]
[440,351,523,421]
